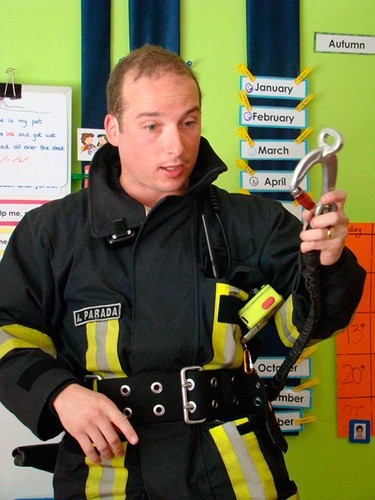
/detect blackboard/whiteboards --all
[0,83,72,261]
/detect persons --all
[0,45,366,500]
[354,425,365,439]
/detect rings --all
[327,227,331,239]
